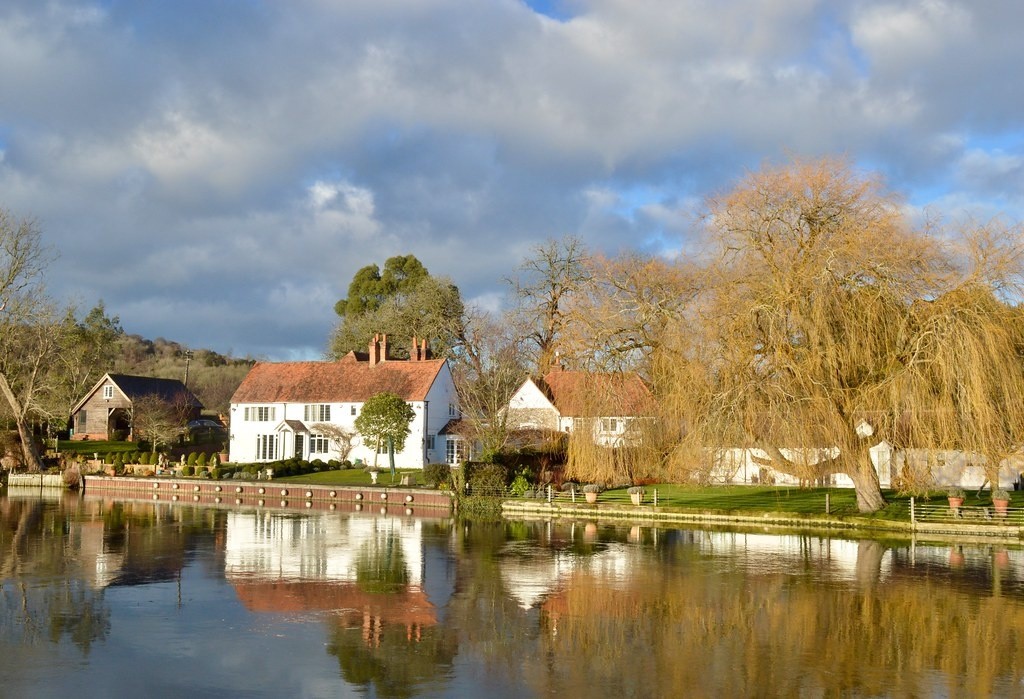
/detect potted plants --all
[583,485,599,504]
[627,487,647,506]
[947,488,967,509]
[991,490,1011,515]
[110,466,116,477]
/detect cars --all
[180,419,224,429]
